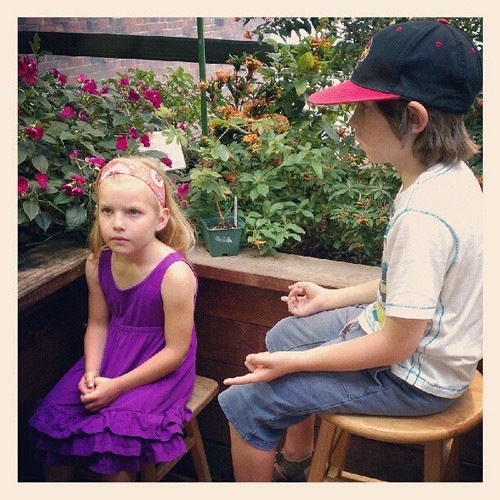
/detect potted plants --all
[189,167,246,258]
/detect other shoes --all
[274,449,313,482]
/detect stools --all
[306,369,483,483]
[61,375,220,482]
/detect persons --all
[27,157,198,482]
[219,19,483,483]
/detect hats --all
[308,18,483,116]
